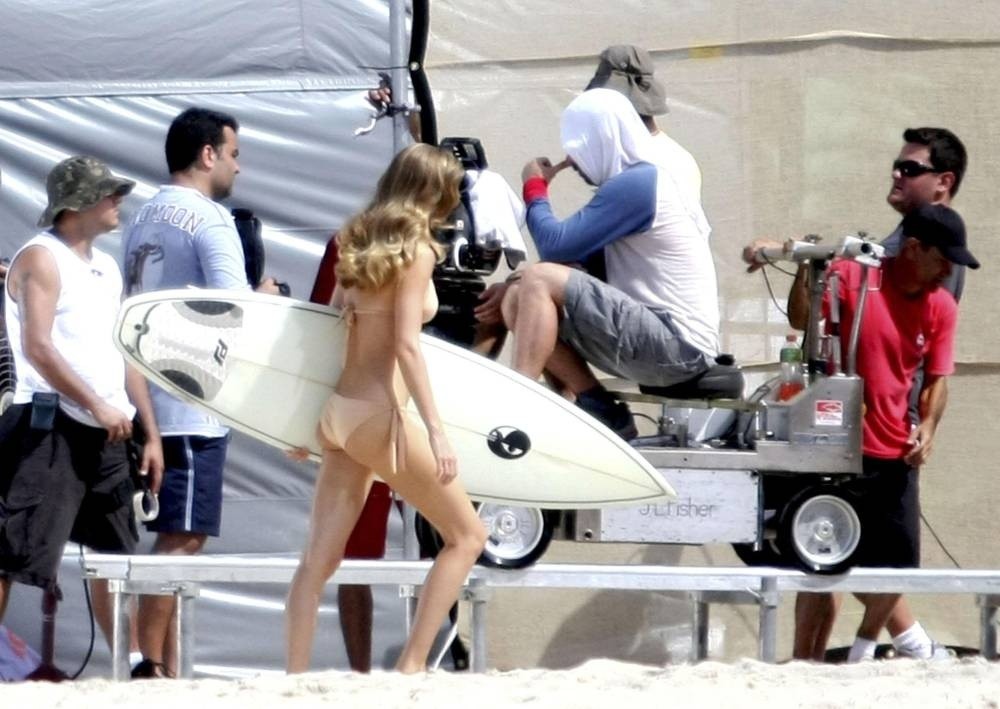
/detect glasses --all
[893,160,941,177]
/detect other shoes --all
[131,659,176,678]
[895,640,956,660]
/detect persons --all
[532,43,712,403]
[787,203,981,663]
[791,127,969,665]
[285,143,486,673]
[122,104,290,680]
[0,155,164,681]
[420,168,528,361]
[500,88,721,443]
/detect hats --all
[902,203,979,269]
[584,45,669,116]
[37,154,136,228]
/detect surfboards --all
[114,287,678,512]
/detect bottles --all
[776,335,804,402]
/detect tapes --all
[133,488,161,522]
